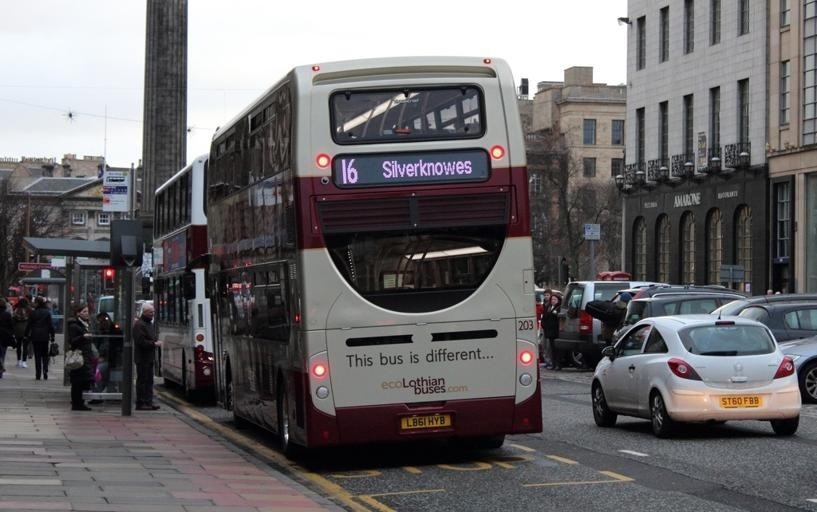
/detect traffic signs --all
[18,263,57,271]
[584,223,600,240]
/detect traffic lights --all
[104,269,117,291]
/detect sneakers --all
[17,359,21,367]
[21,361,26,368]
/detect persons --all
[133,303,162,410]
[548,294,564,372]
[0,290,121,412]
[542,289,550,364]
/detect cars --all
[713,293,817,401]
[591,314,802,436]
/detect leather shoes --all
[43,369,47,379]
[151,405,160,409]
[71,404,91,411]
[135,402,152,410]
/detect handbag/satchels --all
[64,336,84,370]
[49,342,58,356]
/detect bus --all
[598,270,631,281]
[207,56,543,458]
[150,154,209,400]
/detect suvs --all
[535,275,748,366]
